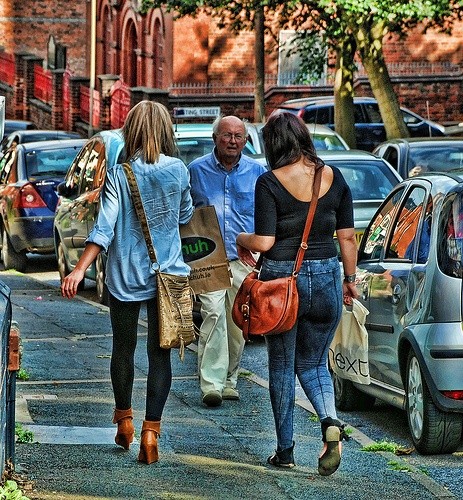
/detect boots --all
[139,422,161,464]
[113,409,133,451]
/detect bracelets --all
[344,274,356,283]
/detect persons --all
[185,115,267,406]
[62,101,198,465]
[235,112,358,475]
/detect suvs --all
[264,95,463,153]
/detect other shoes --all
[268,443,295,467]
[318,419,346,476]
[203,392,222,407]
[222,387,238,399]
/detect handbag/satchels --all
[156,273,195,350]
[329,296,371,386]
[179,205,233,295]
[232,272,298,343]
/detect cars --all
[0,120,463,337]
[328,172,463,455]
